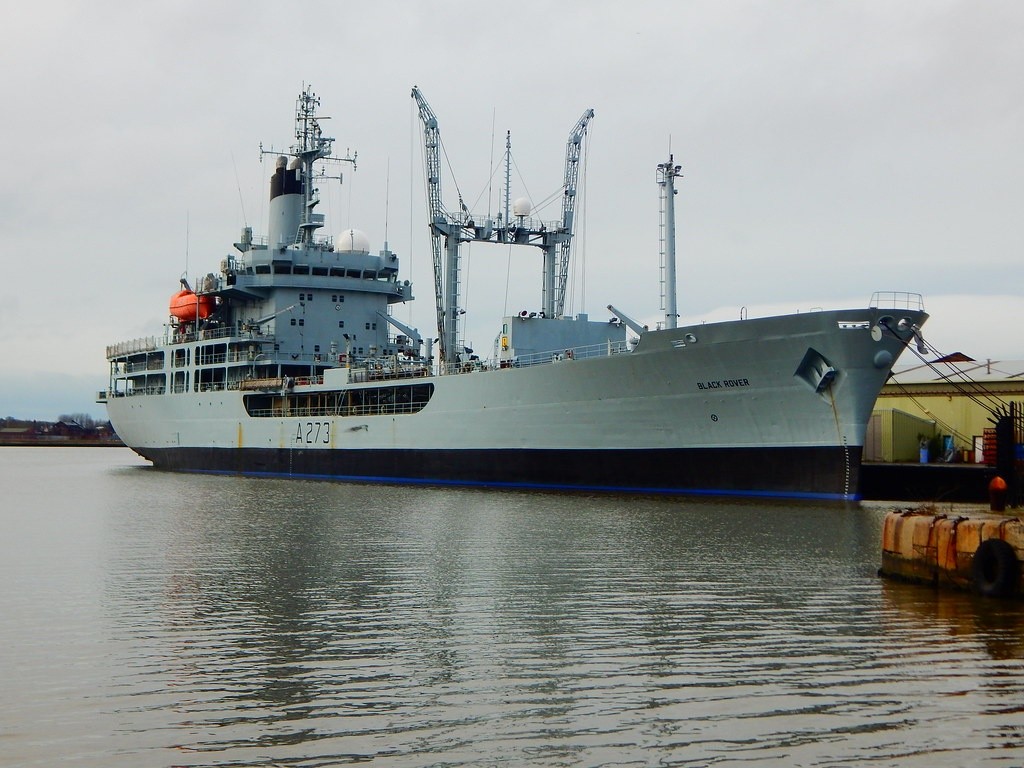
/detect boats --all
[94,78,928,507]
